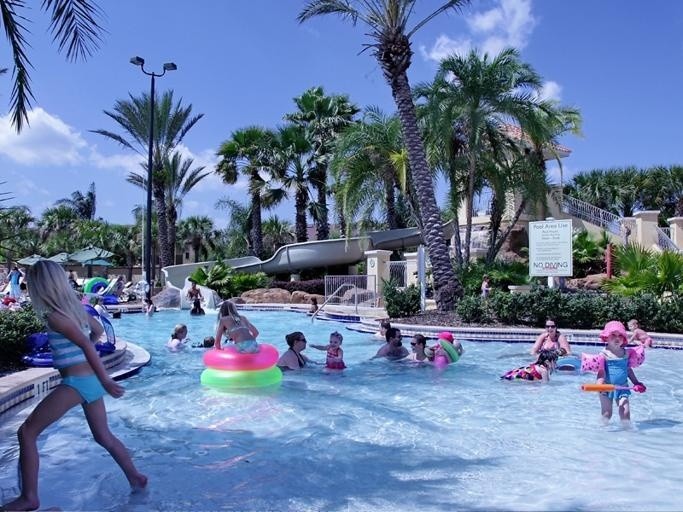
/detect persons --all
[309,297,318,312]
[117,277,124,296]
[214,301,260,353]
[399,334,430,361]
[531,318,572,357]
[551,353,558,370]
[69,270,74,285]
[144,299,154,316]
[94,296,107,316]
[626,319,653,348]
[190,299,205,316]
[377,328,410,358]
[7,267,23,298]
[595,321,646,419]
[168,324,187,352]
[187,281,202,300]
[20,283,29,302]
[276,332,314,372]
[498,349,553,384]
[480,275,495,297]
[309,331,346,369]
[379,323,391,341]
[434,330,454,369]
[0,260,147,512]
[193,335,215,348]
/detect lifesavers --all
[200,366,282,388]
[102,296,119,305]
[554,355,580,371]
[203,344,279,370]
[19,351,54,368]
[84,277,110,303]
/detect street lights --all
[130,55,177,305]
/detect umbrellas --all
[47,251,81,272]
[68,243,119,278]
[17,253,48,277]
[70,258,113,278]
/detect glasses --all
[545,325,556,328]
[395,335,402,339]
[410,342,421,346]
[297,339,306,343]
[12,269,17,270]
